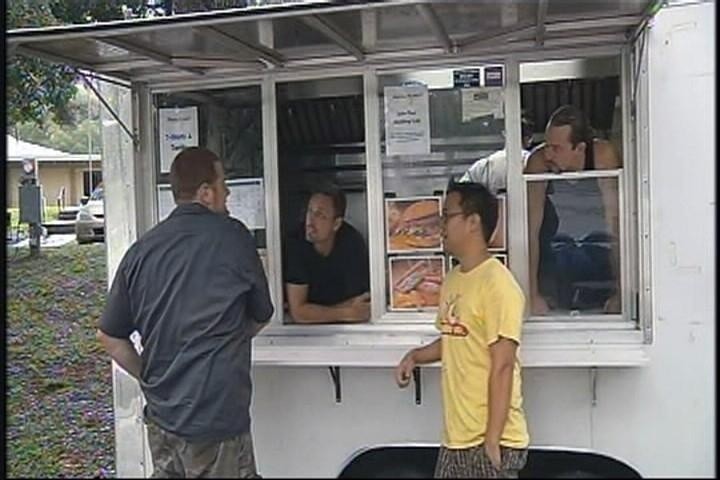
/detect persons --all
[283,183,371,323]
[459,107,535,194]
[96,146,274,479]
[523,105,622,315]
[396,172,531,479]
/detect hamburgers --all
[402,201,440,247]
[388,207,403,240]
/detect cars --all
[75,183,106,245]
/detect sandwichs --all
[418,266,442,292]
[394,261,429,293]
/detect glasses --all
[441,211,463,222]
